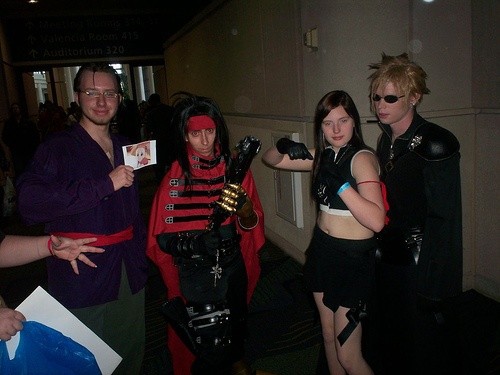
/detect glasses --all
[369,93,406,103]
[79,90,119,99]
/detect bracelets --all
[238,209,258,230]
[48,238,55,256]
[337,181,350,194]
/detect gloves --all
[177,230,242,264]
[276,138,313,160]
[214,184,258,229]
[321,169,347,194]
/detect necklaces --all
[99,136,113,159]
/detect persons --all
[0,94,175,186]
[136,147,148,167]
[145,92,271,375]
[262,89,384,375]
[0,231,106,342]
[366,53,462,375]
[15,64,149,375]
